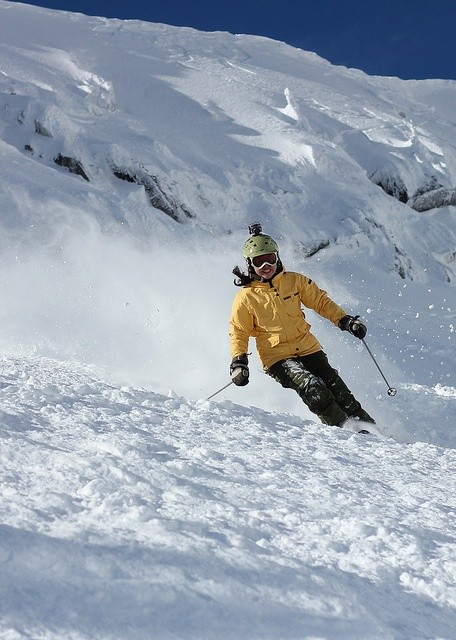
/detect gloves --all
[230,356,249,386]
[339,315,367,339]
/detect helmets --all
[243,235,278,258]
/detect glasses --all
[250,253,279,269]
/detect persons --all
[228,224,376,435]
